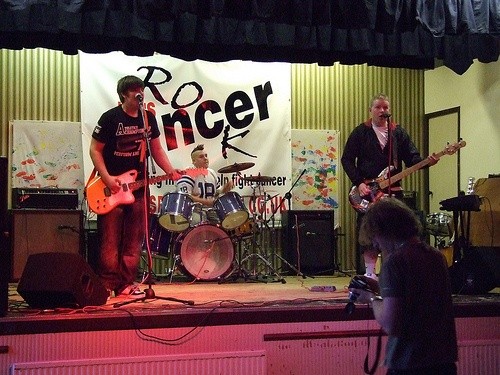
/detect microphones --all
[58,225,71,229]
[382,112,391,118]
[135,92,143,101]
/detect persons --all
[90,75,183,296]
[175,144,233,206]
[341,95,440,279]
[349,198,459,375]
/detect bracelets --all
[371,295,383,303]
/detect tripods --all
[113,105,196,306]
[135,197,308,283]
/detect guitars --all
[84,164,211,215]
[346,140,467,215]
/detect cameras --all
[347,276,371,304]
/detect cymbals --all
[242,176,276,183]
[216,162,256,174]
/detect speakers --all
[448,254,491,295]
[7,207,84,282]
[356,204,418,274]
[16,252,109,308]
[287,210,335,276]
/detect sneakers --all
[107,287,112,301]
[116,283,146,299]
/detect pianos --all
[439,193,482,265]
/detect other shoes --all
[363,273,377,281]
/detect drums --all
[171,220,238,281]
[141,208,175,259]
[234,217,260,240]
[156,192,194,233]
[212,191,250,228]
